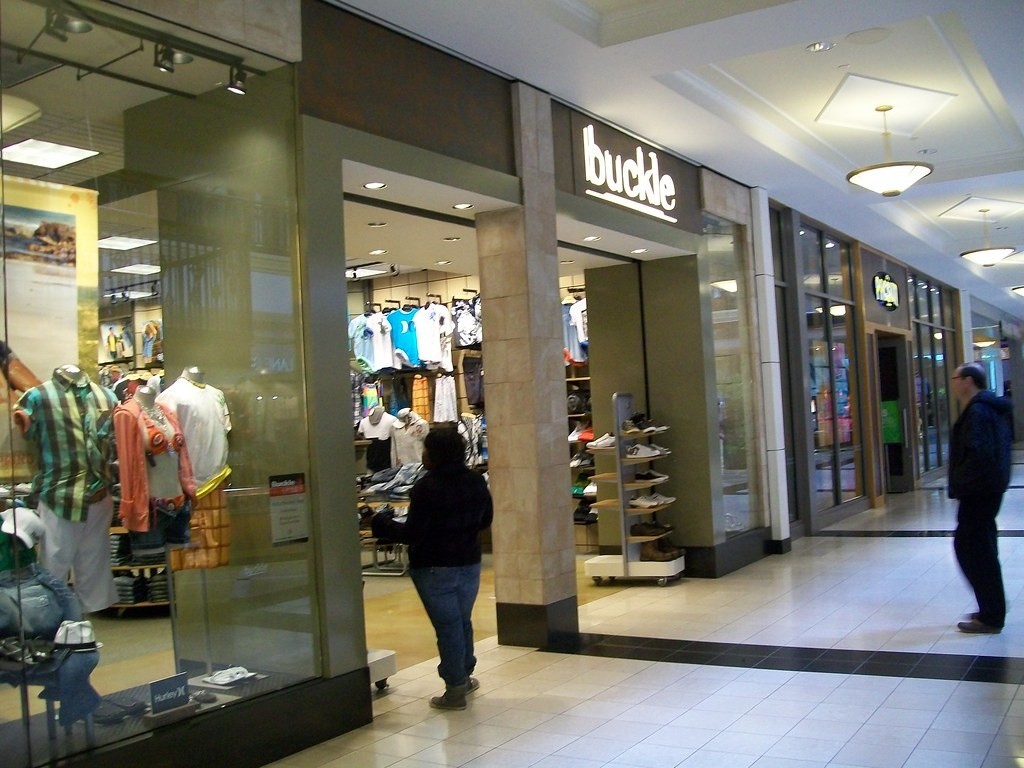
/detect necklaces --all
[176,376,209,389]
[130,396,167,425]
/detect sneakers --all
[570,448,594,468]
[574,501,598,523]
[583,481,598,493]
[620,414,671,433]
[634,469,670,482]
[566,421,589,441]
[626,443,673,459]
[578,427,594,441]
[586,432,616,449]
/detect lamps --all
[957,207,1016,267]
[843,102,933,201]
[813,306,824,314]
[154,43,179,74]
[111,279,160,303]
[224,64,252,96]
[830,276,846,317]
[1011,283,1024,296]
[974,333,998,346]
[711,276,739,293]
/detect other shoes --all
[958,612,1002,633]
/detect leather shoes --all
[640,541,686,561]
[630,521,673,536]
[464,677,480,695]
[430,684,467,710]
[629,492,677,508]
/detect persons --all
[354,406,428,473]
[1003,380,1017,444]
[15,361,230,612]
[405,428,493,712]
[0,340,44,393]
[948,363,1014,634]
[142,320,164,363]
[107,326,133,359]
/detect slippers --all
[0,635,55,670]
[202,667,258,686]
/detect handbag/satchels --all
[567,388,592,415]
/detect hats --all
[369,407,383,425]
[109,366,121,372]
[0,506,45,549]
[103,367,109,375]
[159,370,165,377]
[393,407,421,428]
[152,341,163,355]
[51,620,103,651]
[141,372,154,381]
[125,380,140,394]
[128,362,134,369]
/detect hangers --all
[362,292,439,314]
[560,286,576,305]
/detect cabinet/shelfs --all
[564,376,599,547]
[108,523,170,620]
[584,390,694,587]
[357,501,409,576]
